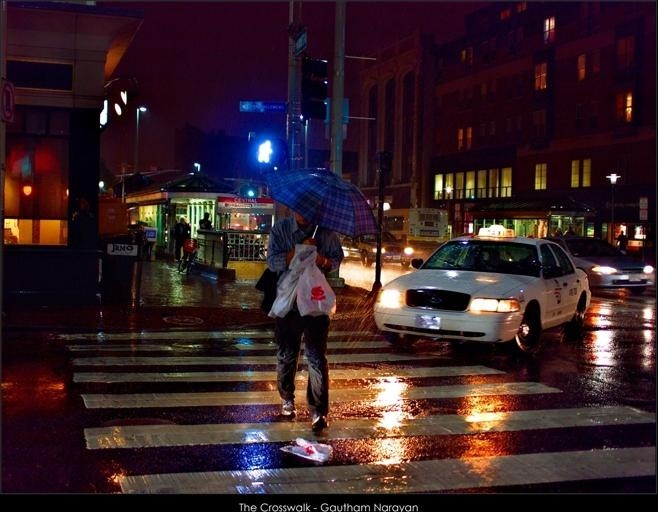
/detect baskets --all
[183,239,197,253]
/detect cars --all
[372,221,594,360]
[337,229,415,269]
[537,234,656,297]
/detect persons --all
[199,213,212,230]
[266,210,344,434]
[174,217,190,263]
[615,231,626,249]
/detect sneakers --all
[279,398,297,417]
[309,413,329,434]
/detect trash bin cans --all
[101,254,143,306]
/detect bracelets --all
[288,251,293,260]
[322,257,327,268]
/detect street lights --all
[604,172,623,249]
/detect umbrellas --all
[263,165,381,239]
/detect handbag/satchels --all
[279,437,334,464]
[267,246,337,319]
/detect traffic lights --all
[301,51,330,123]
